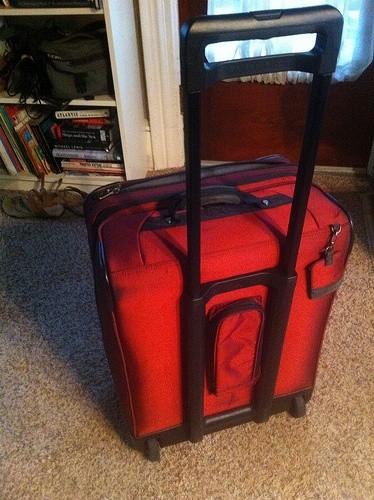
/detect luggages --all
[81,4,355,464]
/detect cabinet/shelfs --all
[0,0,154,198]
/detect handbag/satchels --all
[37,15,109,101]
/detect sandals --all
[2,187,65,220]
[54,182,86,218]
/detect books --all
[0,102,127,183]
